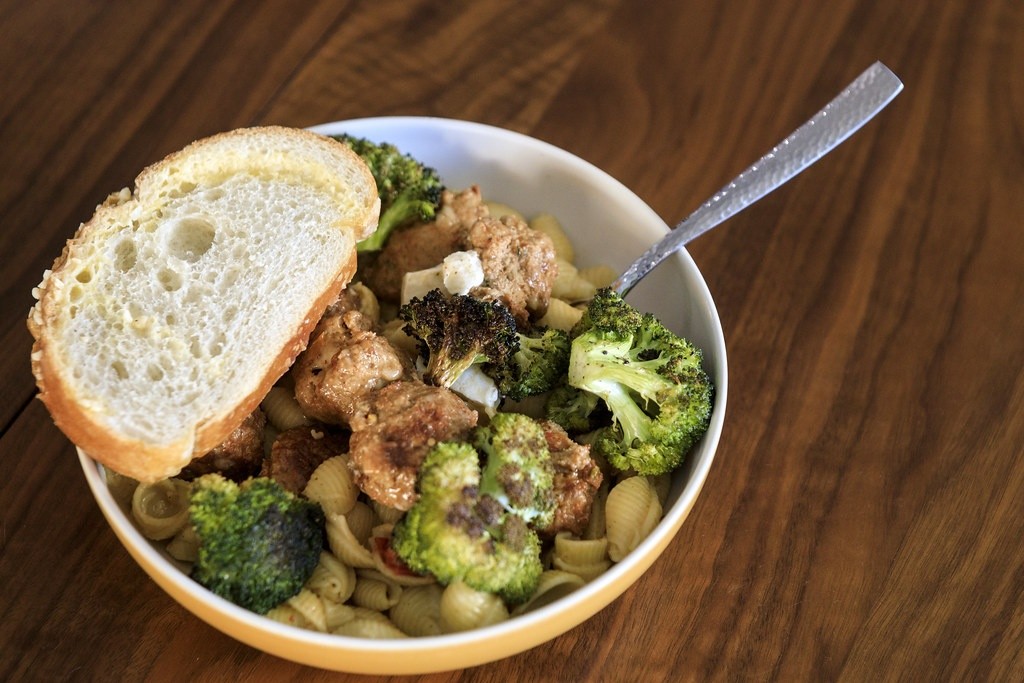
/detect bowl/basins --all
[75,116,728,675]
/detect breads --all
[27,127,381,490]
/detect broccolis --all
[184,472,327,618]
[326,133,445,252]
[393,284,714,593]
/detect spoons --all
[497,61,905,399]
